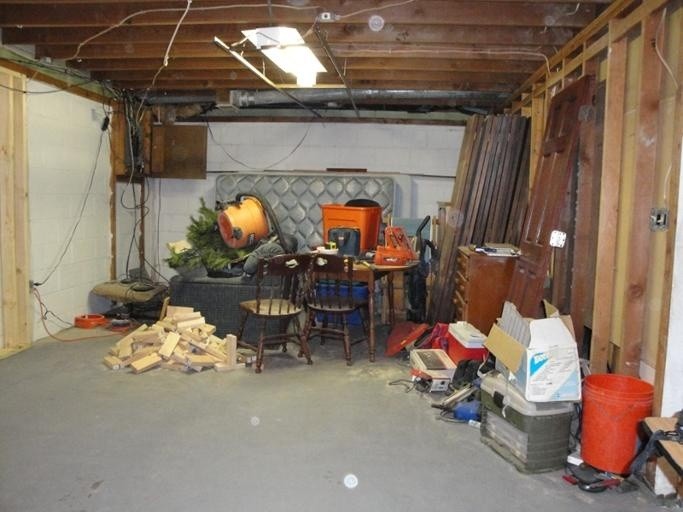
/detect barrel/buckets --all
[581,374,654,474]
[220,198,269,248]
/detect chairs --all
[167,230,371,376]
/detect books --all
[484,242,513,249]
[482,250,518,256]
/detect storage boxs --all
[319,202,382,253]
[412,320,489,393]
[478,299,583,477]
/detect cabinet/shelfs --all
[450,246,516,335]
